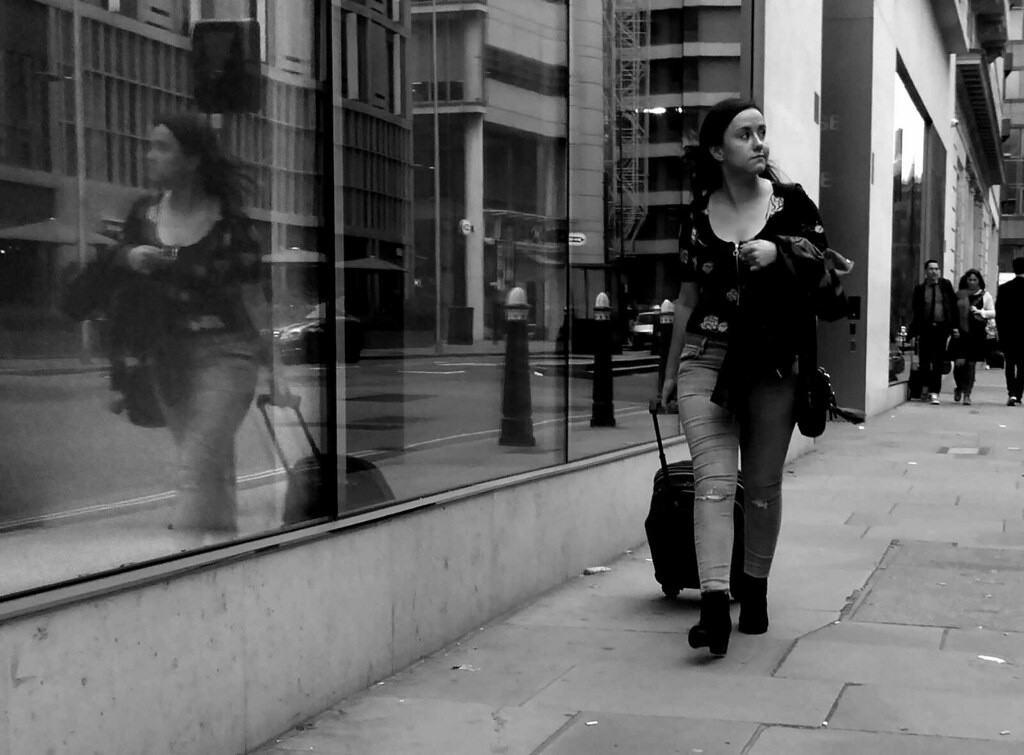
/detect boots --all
[739,574,769,635]
[687,589,732,654]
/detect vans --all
[275,316,367,367]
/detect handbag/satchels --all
[968,289,989,329]
[794,366,837,437]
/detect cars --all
[633,312,658,344]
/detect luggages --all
[643,393,747,602]
[990,338,1005,369]
[906,335,923,401]
[256,390,397,523]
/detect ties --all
[927,283,937,328]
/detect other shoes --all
[963,394,971,405]
[920,386,929,401]
[985,365,990,370]
[1007,395,1018,406]
[954,388,961,401]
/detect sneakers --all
[929,392,941,405]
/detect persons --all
[906,260,960,401]
[110,114,291,529]
[995,257,1024,406]
[662,97,828,657]
[984,318,999,370]
[948,269,995,405]
